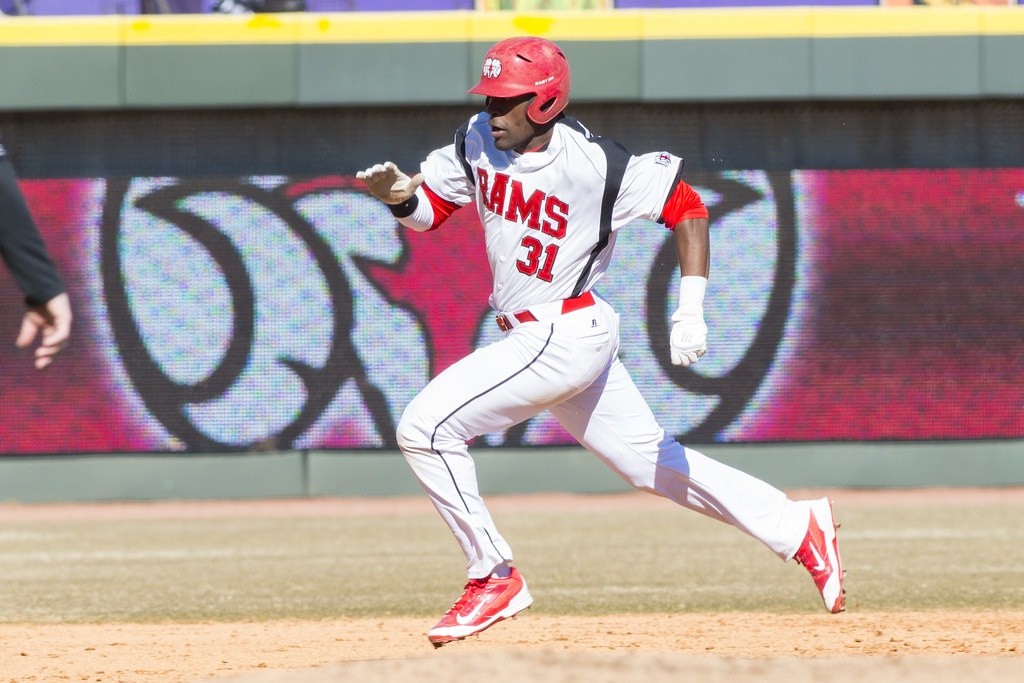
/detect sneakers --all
[793,497,846,613]
[427,566,533,647]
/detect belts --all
[496,291,596,332]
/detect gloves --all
[671,300,708,366]
[355,162,424,205]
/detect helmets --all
[466,36,570,124]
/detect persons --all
[0,141,73,369]
[356,36,848,644]
